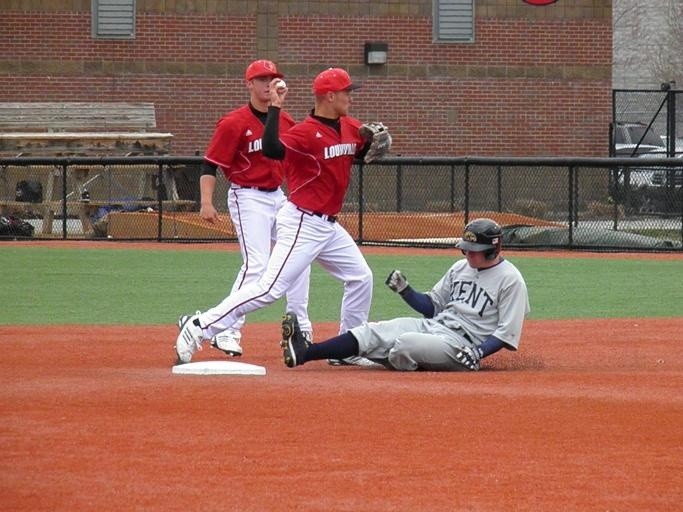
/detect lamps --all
[365,41,387,66]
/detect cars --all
[614,121,683,188]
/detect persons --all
[279,218,531,371]
[173,67,393,366]
[199,59,313,357]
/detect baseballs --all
[274,77,285,92]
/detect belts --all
[241,184,280,193]
[457,327,473,344]
[314,211,339,222]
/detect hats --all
[311,66,364,98]
[245,59,284,81]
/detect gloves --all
[385,269,409,293]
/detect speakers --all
[363,43,388,66]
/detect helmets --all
[455,218,503,253]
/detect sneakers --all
[456,344,481,371]
[210,328,243,358]
[173,315,205,363]
[327,355,377,366]
[280,310,307,368]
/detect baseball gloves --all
[360,124,390,164]
